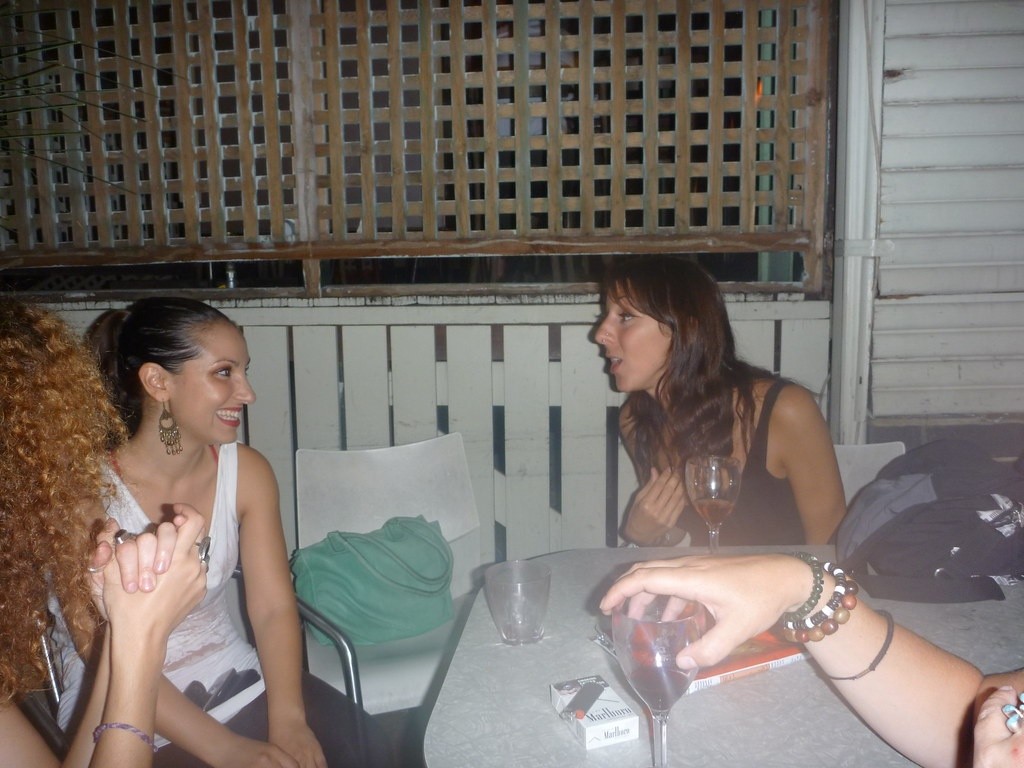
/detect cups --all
[486,561,550,643]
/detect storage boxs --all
[550,674,642,750]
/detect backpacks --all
[835,438,1024,603]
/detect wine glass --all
[685,453,743,556]
[612,594,705,768]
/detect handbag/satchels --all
[291,514,454,647]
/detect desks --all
[415,544,1024,768]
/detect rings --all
[195,536,212,560]
[87,565,103,573]
[114,528,133,545]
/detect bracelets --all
[825,609,895,681]
[778,552,858,645]
[92,723,159,755]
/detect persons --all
[591,253,847,547]
[597,550,1024,768]
[0,293,396,768]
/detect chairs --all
[295,433,485,768]
[40,566,364,768]
[833,440,907,507]
